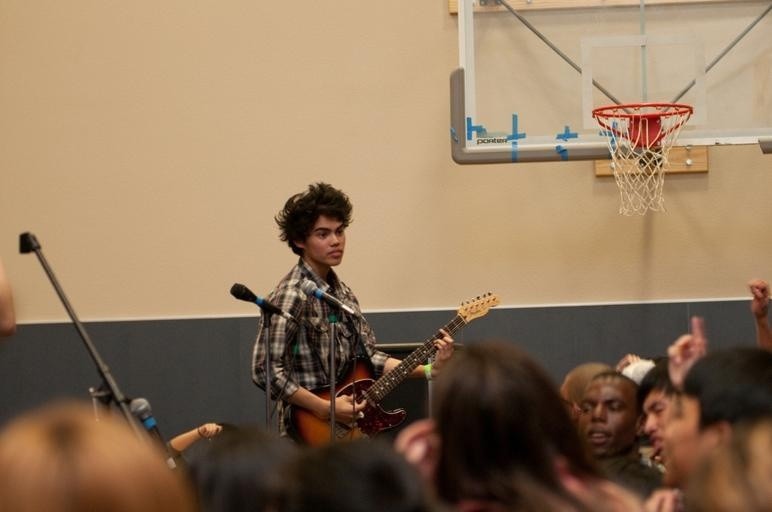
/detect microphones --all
[129,397,176,467]
[301,280,359,322]
[230,283,297,326]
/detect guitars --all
[285,292,500,451]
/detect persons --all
[252,181,456,448]
[0,260,771,511]
